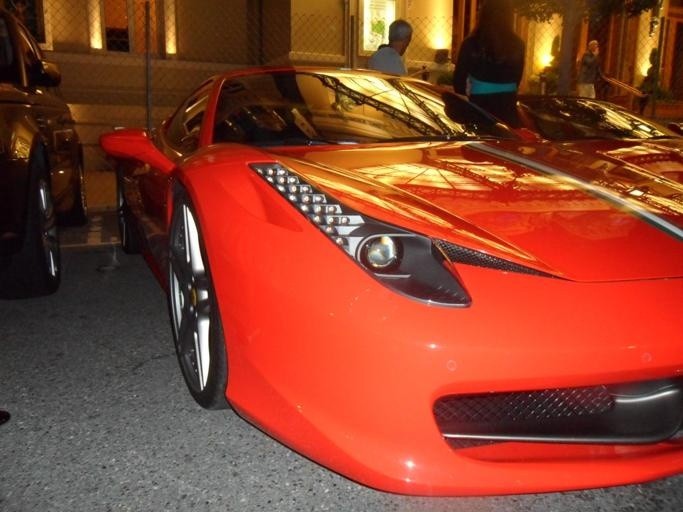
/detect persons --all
[575,40,603,99]
[452,0,524,121]
[365,19,412,77]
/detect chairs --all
[214,103,324,143]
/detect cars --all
[0,2,86,295]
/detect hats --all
[588,41,600,45]
